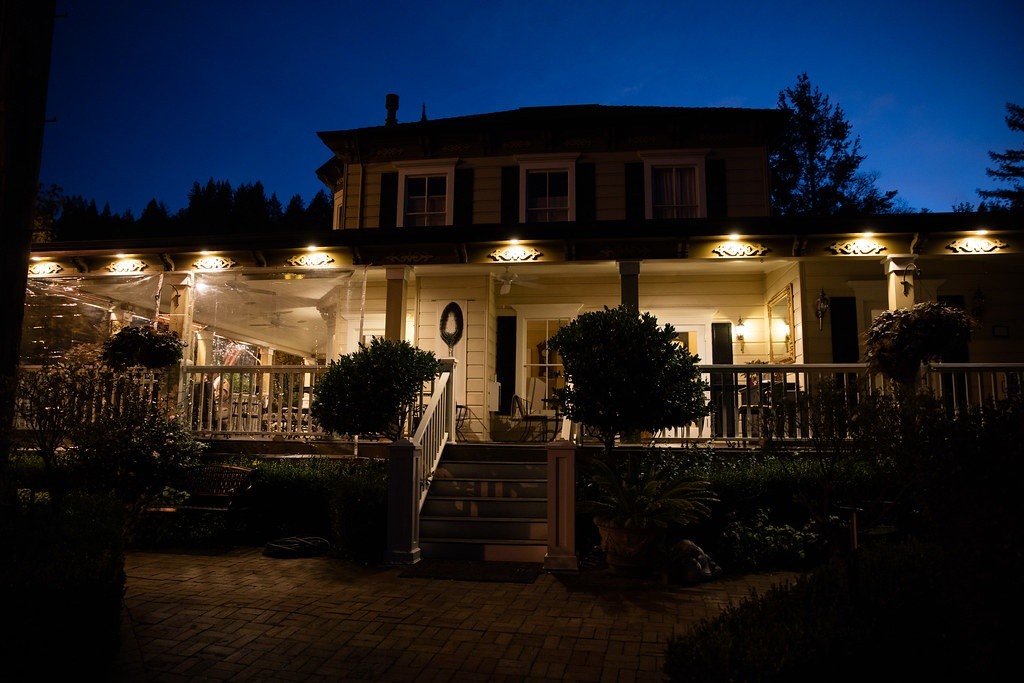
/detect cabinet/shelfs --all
[740,383,797,438]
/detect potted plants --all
[579,452,721,576]
[741,360,768,386]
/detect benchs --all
[142,464,258,554]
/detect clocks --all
[536,340,552,376]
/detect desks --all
[542,398,584,441]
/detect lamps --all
[155,283,181,306]
[815,288,829,331]
[735,316,744,341]
[901,263,921,296]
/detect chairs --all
[738,381,782,437]
[456,405,468,443]
[190,381,229,431]
[511,395,548,443]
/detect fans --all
[492,265,540,295]
[248,312,297,330]
[204,274,277,297]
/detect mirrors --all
[767,283,796,364]
